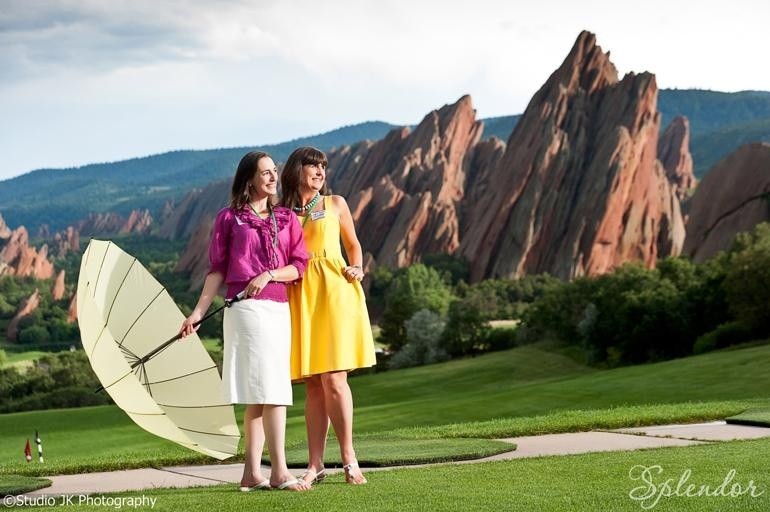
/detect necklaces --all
[293,193,320,210]
[247,202,277,249]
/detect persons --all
[281,147,378,485]
[177,152,315,492]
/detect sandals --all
[343,460,368,486]
[297,468,327,485]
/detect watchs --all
[267,269,277,283]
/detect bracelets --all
[352,265,362,269]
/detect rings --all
[251,292,256,294]
[352,271,357,276]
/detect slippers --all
[239,478,271,492]
[269,477,314,492]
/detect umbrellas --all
[76,238,249,461]
